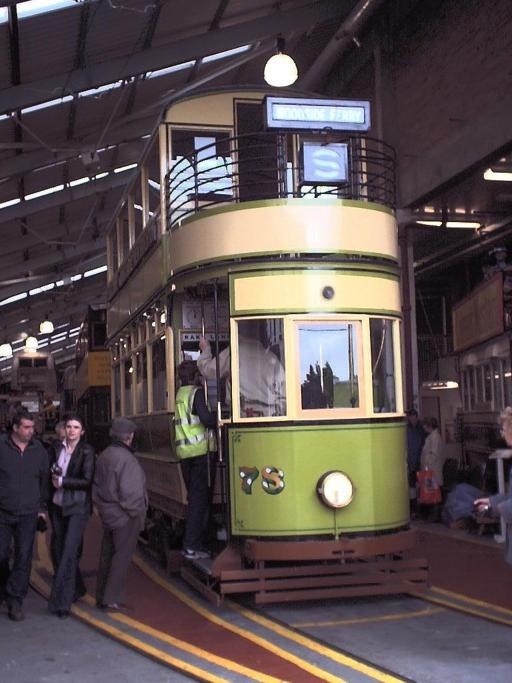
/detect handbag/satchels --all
[416,470,442,504]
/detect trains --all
[61,84,428,610]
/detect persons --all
[404,405,511,565]
[44,412,97,618]
[48,418,97,580]
[90,415,150,614]
[196,318,286,418]
[0,409,52,621]
[168,358,225,561]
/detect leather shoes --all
[96,602,128,612]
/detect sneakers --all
[3,589,25,621]
[181,548,211,558]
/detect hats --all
[113,417,137,432]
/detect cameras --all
[51,463,63,476]
[36,515,48,533]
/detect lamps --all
[263,37,298,89]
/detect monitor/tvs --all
[302,141,351,186]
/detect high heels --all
[73,587,87,603]
[58,611,69,620]
